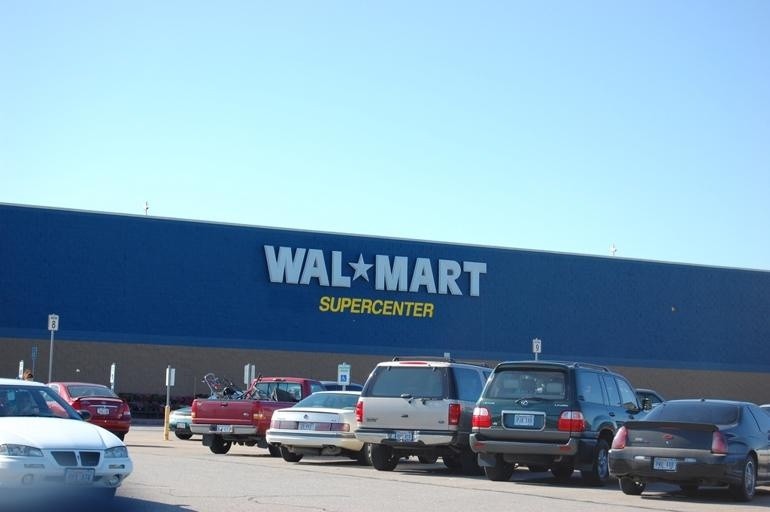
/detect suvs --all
[470,358,647,488]
[353,354,496,475]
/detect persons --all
[23,368,35,381]
[7,391,40,416]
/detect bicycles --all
[220,374,266,402]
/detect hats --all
[0,378,133,504]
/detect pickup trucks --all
[190,376,326,456]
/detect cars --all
[634,389,665,410]
[266,390,375,466]
[606,398,770,503]
[0,378,133,504]
[318,380,363,392]
[170,392,242,440]
